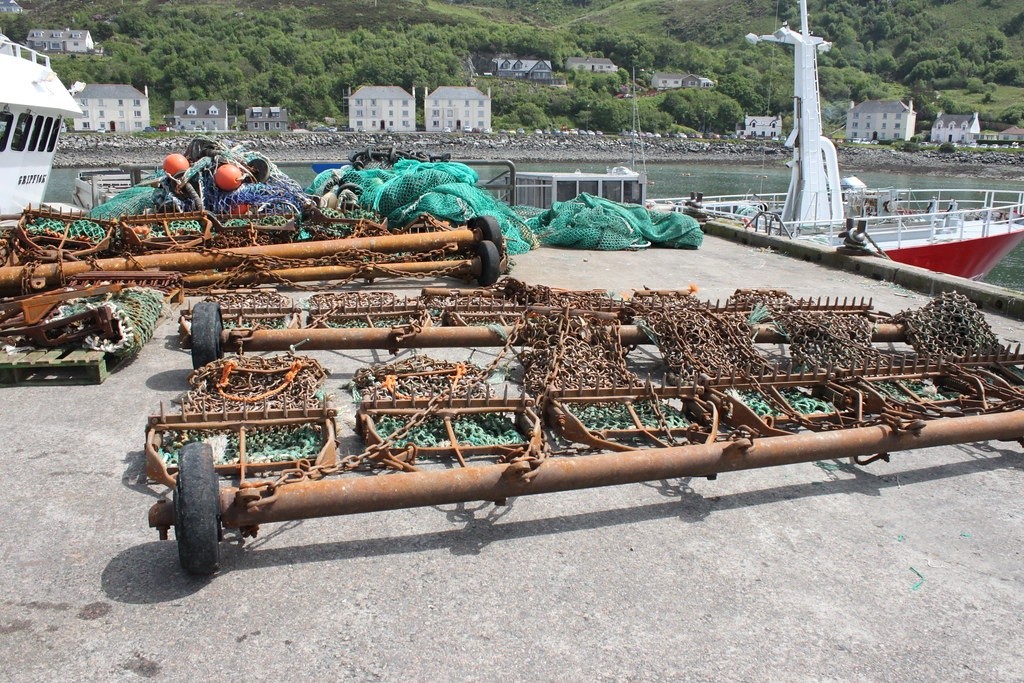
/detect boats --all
[644,0,1024,282]
[0,32,85,226]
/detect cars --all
[387,127,395,133]
[97,128,106,133]
[619,131,738,140]
[358,127,367,133]
[920,141,1024,149]
[313,127,331,132]
[739,135,747,140]
[755,135,764,141]
[779,136,787,141]
[143,123,207,133]
[771,136,779,141]
[829,138,880,145]
[440,126,606,136]
